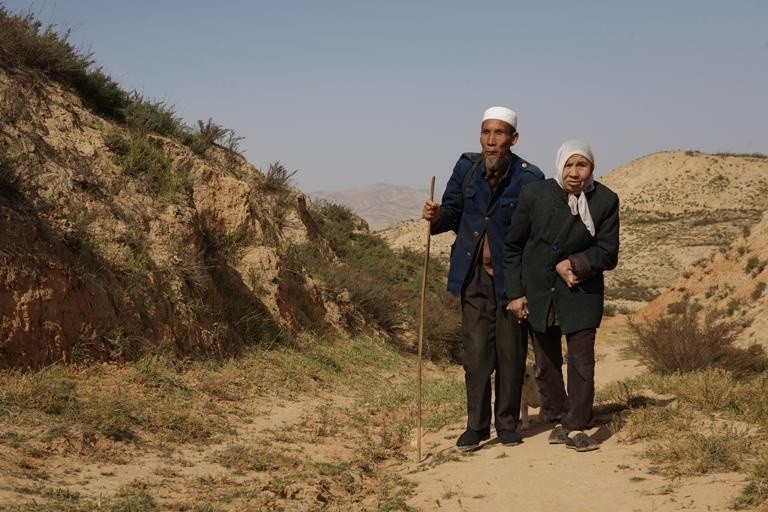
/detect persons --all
[423,107,545,450]
[502,139,619,452]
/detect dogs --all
[519,362,544,432]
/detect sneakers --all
[548,426,571,444]
[496,429,523,447]
[456,427,491,449]
[566,433,600,452]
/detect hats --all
[482,106,518,130]
